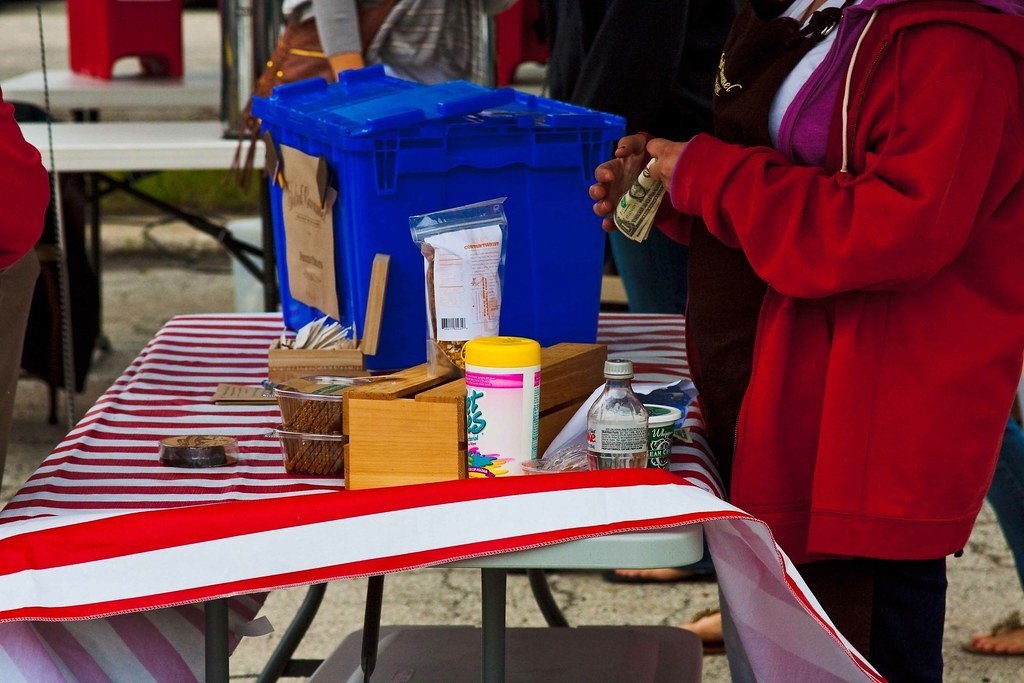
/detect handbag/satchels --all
[241,0,399,141]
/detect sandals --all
[675,600,727,654]
[961,610,1024,655]
[602,564,716,582]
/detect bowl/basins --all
[270,373,372,476]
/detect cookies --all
[283,400,345,477]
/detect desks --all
[0,313,885,683]
[19,120,280,425]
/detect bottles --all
[588,359,646,471]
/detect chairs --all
[6,96,111,420]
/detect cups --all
[461,336,541,479]
[641,404,681,471]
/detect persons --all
[544,0,1024,683]
[259,0,519,312]
[0,89,50,487]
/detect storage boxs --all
[306,624,704,683]
[262,375,367,434]
[248,61,626,372]
[342,342,610,491]
[265,423,348,476]
